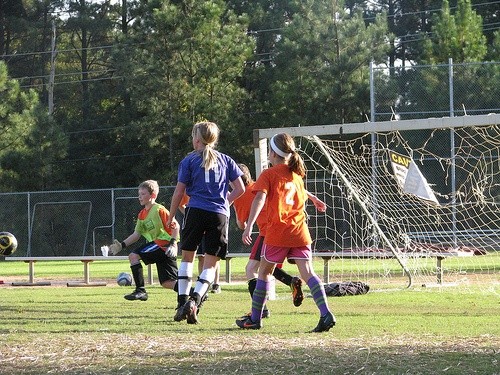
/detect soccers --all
[117,272,133,286]
[0,232,18,256]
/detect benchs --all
[4,252,448,287]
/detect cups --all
[101,247,108,256]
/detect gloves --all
[109,239,122,255]
[165,245,177,258]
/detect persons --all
[236,132,336,332]
[108,179,208,316]
[178,188,222,293]
[228,163,327,319]
[165,119,246,324]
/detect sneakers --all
[123,289,148,301]
[174,304,185,321]
[312,314,335,332]
[291,276,303,307]
[241,310,269,319]
[185,299,198,323]
[212,284,221,293]
[235,317,262,330]
